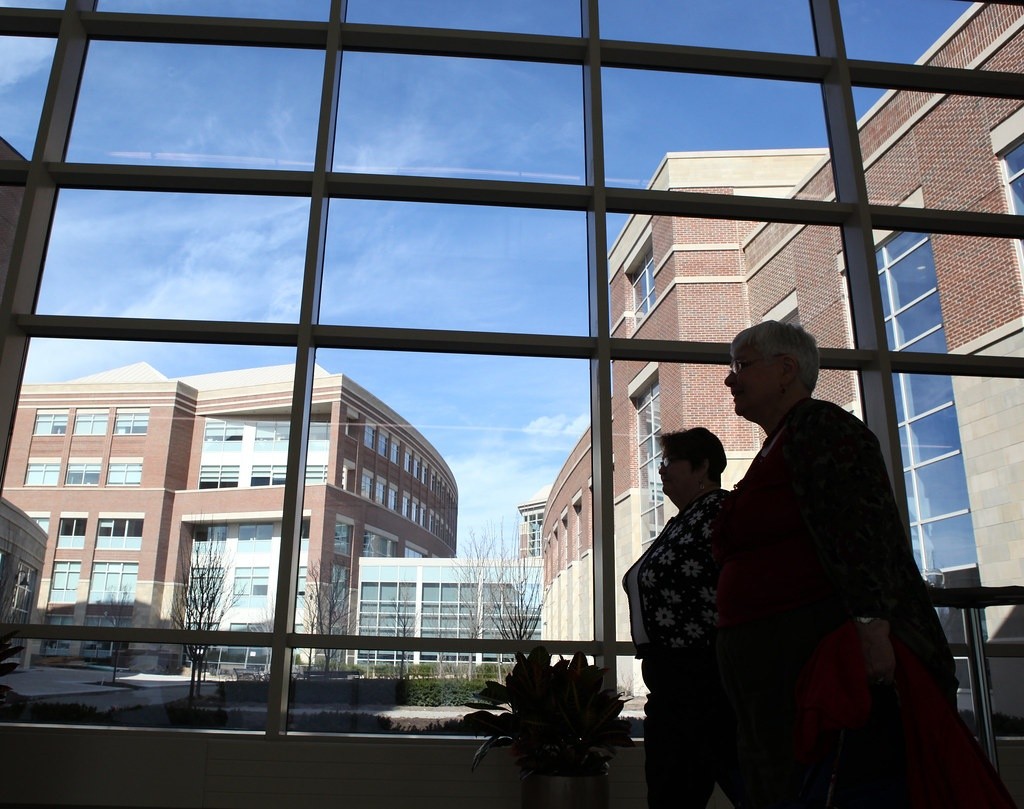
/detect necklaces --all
[763,397,809,449]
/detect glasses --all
[728,355,780,375]
[660,456,687,467]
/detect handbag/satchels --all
[799,678,910,809]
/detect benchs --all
[304,670,360,681]
[234,667,260,681]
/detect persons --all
[622,426,746,809]
[710,322,960,809]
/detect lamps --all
[15,571,29,588]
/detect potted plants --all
[461,645,637,809]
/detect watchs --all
[856,617,877,624]
